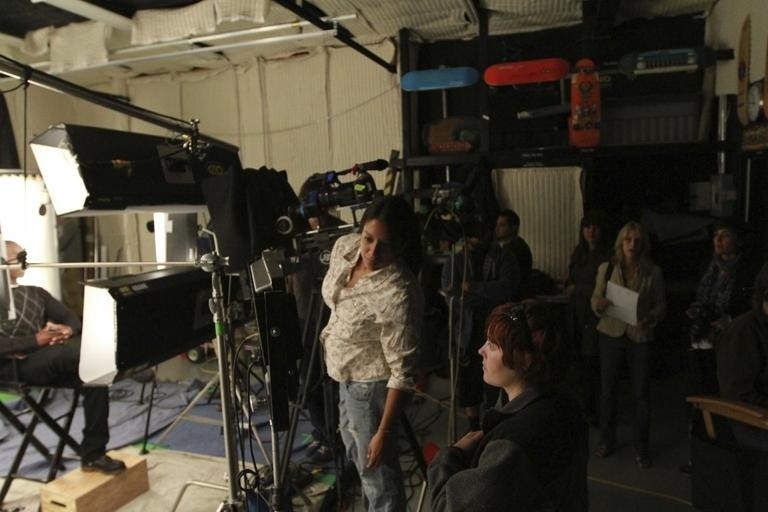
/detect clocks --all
[744,78,768,151]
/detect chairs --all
[681,388,768,449]
[0,346,101,504]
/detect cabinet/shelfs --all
[391,20,735,237]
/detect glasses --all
[506,303,532,347]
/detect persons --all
[441,209,532,434]
[319,196,423,512]
[685,218,768,452]
[569,214,667,470]
[426,299,590,512]
[1,240,127,474]
[299,174,352,232]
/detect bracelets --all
[379,426,388,432]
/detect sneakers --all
[593,442,697,474]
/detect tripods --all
[278,276,429,512]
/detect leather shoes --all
[128,368,154,383]
[81,456,126,475]
